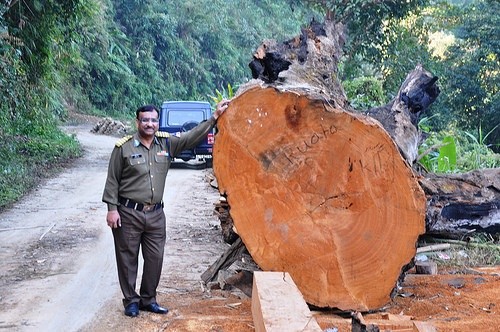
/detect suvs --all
[159,101,214,167]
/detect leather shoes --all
[125,302,139,316]
[139,302,169,313]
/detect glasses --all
[137,118,160,124]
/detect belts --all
[117,195,163,213]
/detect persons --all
[102,98,231,316]
